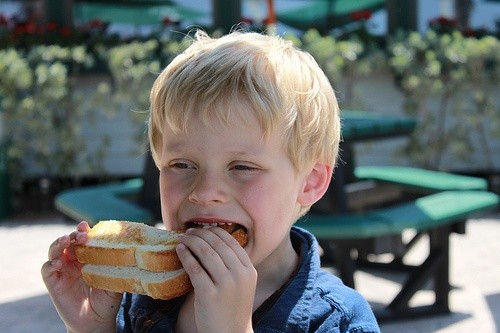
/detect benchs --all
[54,163,500,319]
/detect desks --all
[326,112,417,214]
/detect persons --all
[40,30,381,333]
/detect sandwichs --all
[75,219,248,300]
